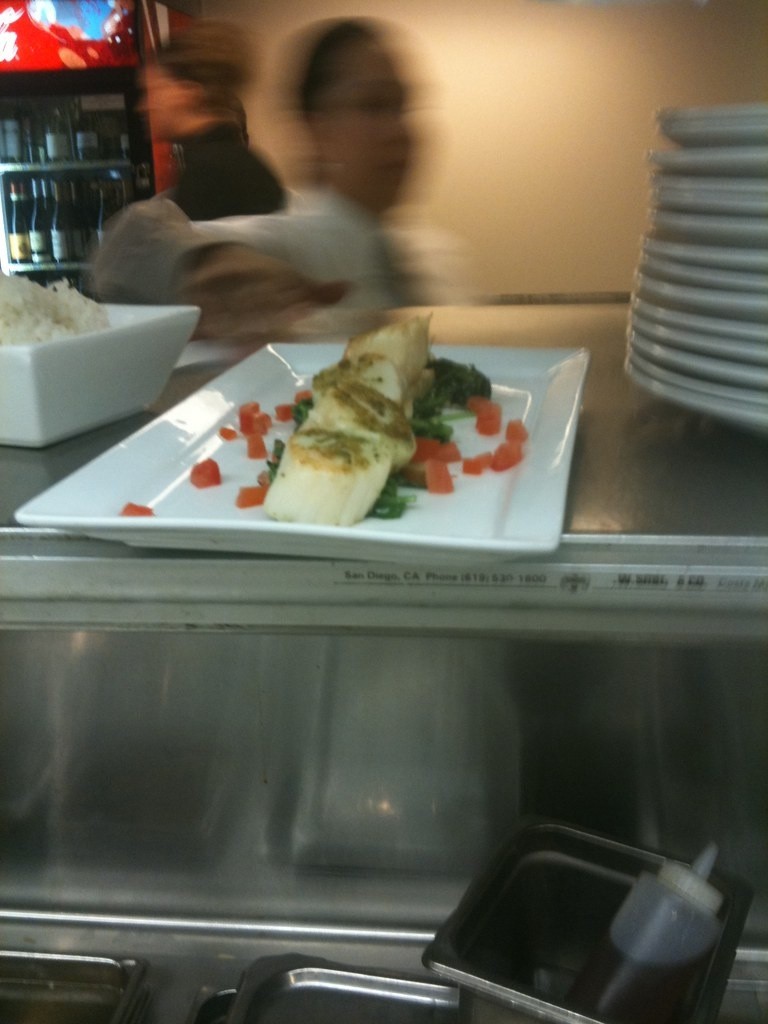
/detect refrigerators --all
[0,0,204,300]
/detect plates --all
[15,341,591,573]
[623,103,768,432]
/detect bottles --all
[8,177,123,263]
[567,840,725,1024]
[0,101,98,164]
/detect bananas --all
[264,310,434,525]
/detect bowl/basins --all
[0,302,201,447]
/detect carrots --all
[119,390,527,519]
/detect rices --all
[0,271,112,348]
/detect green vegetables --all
[264,352,495,519]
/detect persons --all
[148,13,286,221]
[179,15,416,336]
